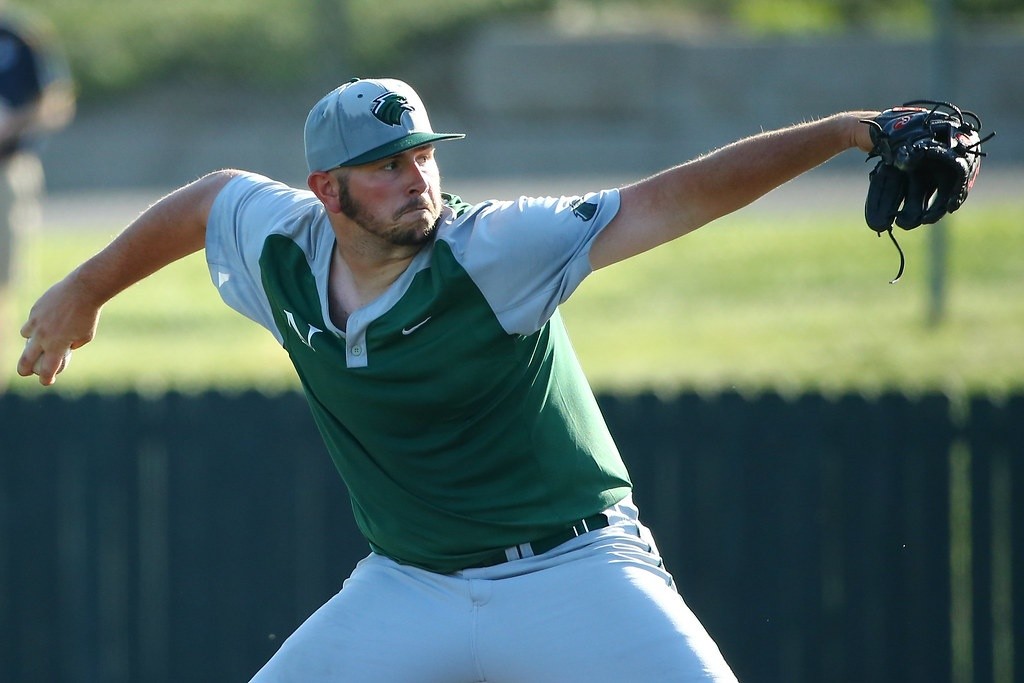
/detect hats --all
[305,77,466,174]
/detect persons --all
[17,78,995,683]
[0,2,78,339]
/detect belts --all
[461,512,609,568]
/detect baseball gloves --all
[863,107,981,233]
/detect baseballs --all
[24,337,72,375]
